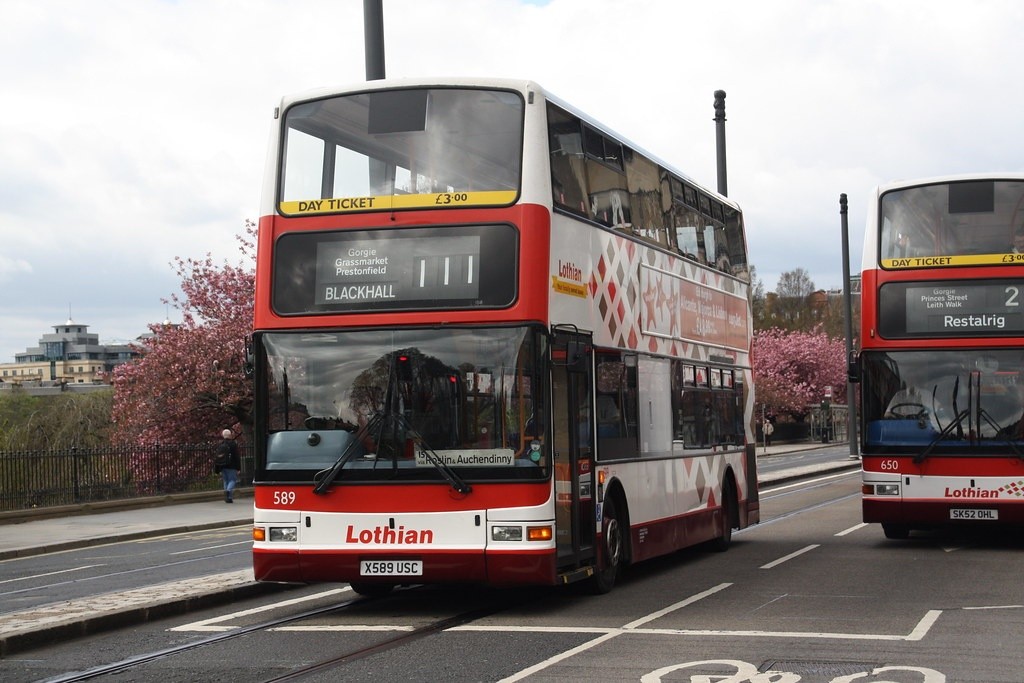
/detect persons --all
[881,368,944,421]
[762,420,774,446]
[213,429,243,503]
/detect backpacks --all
[212,439,233,466]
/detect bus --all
[862,171,1022,549]
[248,76,762,605]
